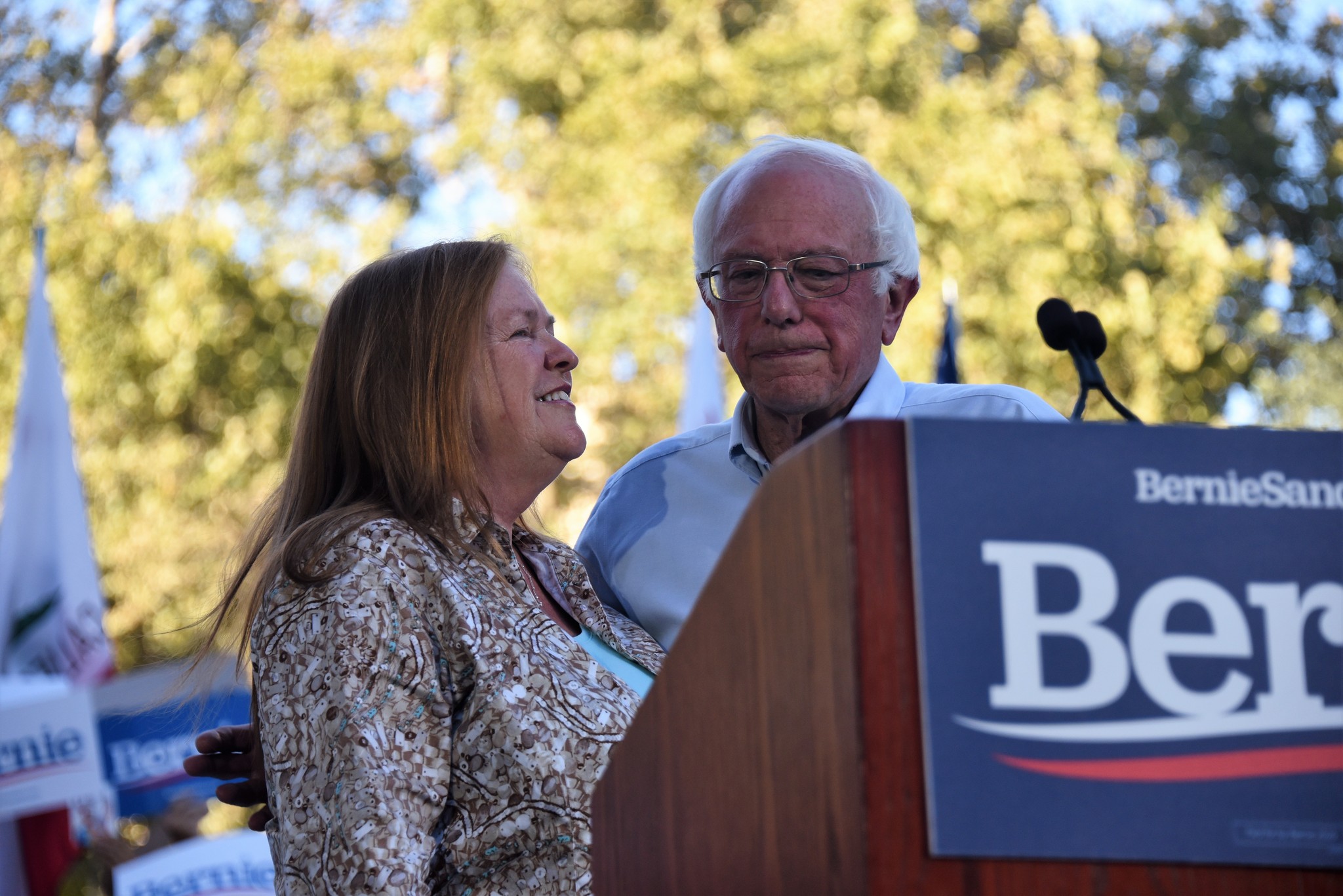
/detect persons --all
[244,239,668,896]
[180,132,1072,896]
[102,795,209,896]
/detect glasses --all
[699,254,893,303]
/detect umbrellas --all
[0,256,118,686]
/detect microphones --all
[1039,299,1140,423]
